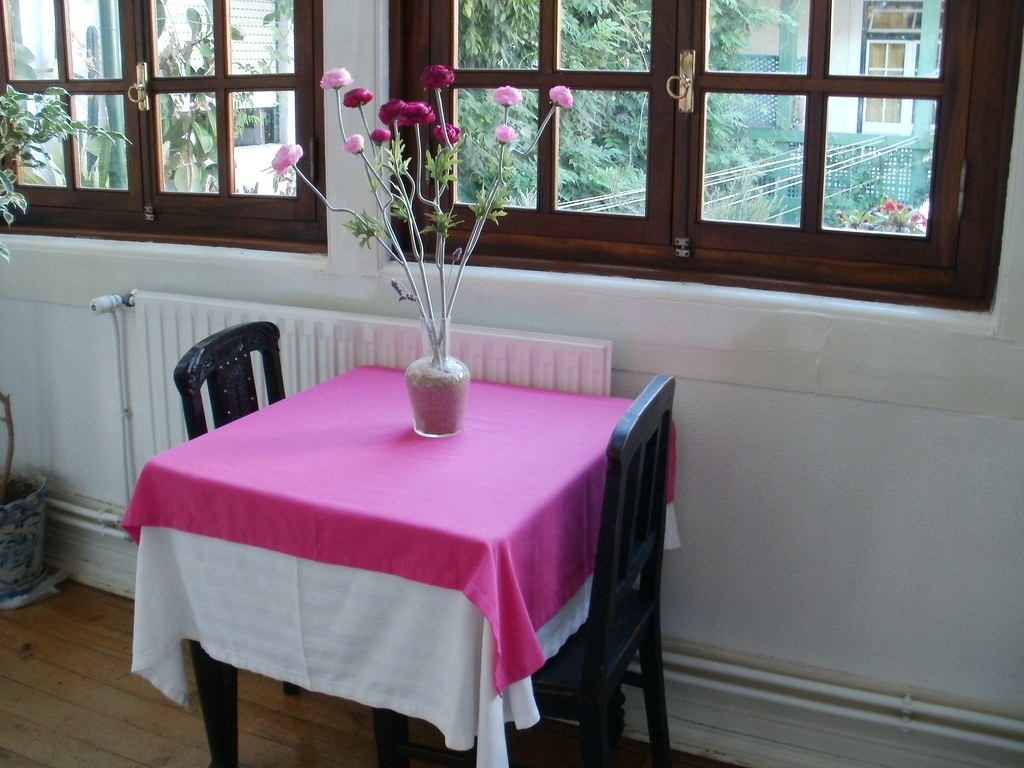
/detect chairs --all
[376,376,677,768]
[174,321,300,695]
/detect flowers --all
[272,64,574,370]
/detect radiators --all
[89,290,613,542]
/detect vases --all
[406,314,469,438]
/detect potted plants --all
[0,84,134,600]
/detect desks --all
[119,366,682,768]
